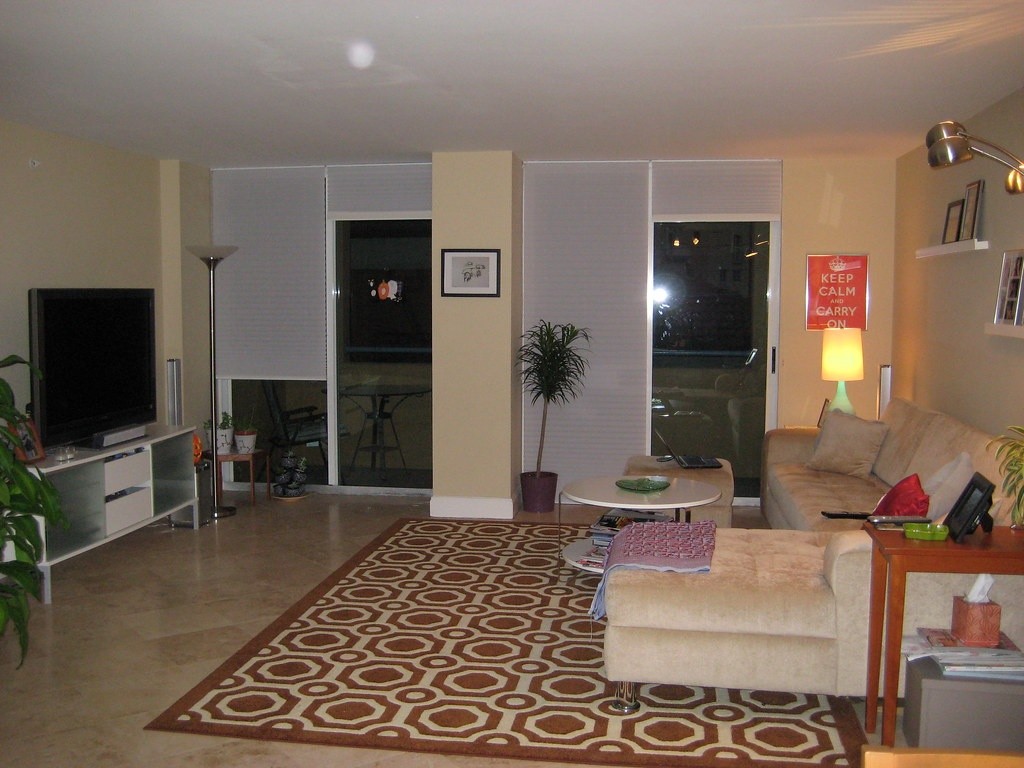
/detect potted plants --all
[205,412,233,455]
[270,449,312,500]
[517,320,591,513]
[234,417,258,453]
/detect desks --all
[321,385,433,482]
[216,452,256,509]
[863,522,1024,751]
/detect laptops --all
[653,427,723,469]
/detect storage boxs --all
[951,596,1001,649]
[903,653,1024,750]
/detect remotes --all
[821,511,873,519]
[867,515,932,522]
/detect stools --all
[622,455,733,530]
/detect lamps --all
[926,121,1023,194]
[822,327,864,412]
[184,244,246,518]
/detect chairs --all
[254,380,349,487]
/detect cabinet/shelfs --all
[2,424,203,605]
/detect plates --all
[903,523,949,540]
[615,479,670,493]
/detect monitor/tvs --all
[26,286,158,452]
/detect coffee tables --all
[558,477,722,572]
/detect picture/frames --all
[993,249,1024,325]
[941,199,964,244]
[943,472,996,542]
[440,248,500,298]
[958,179,984,241]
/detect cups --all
[55,447,66,461]
[66,446,76,459]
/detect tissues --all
[951,573,1001,647]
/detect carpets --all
[143,516,869,768]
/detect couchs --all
[592,528,873,711]
[760,398,1024,530]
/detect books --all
[902,627,1024,680]
[578,509,675,569]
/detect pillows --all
[861,474,929,530]
[805,408,888,480]
[923,452,975,520]
